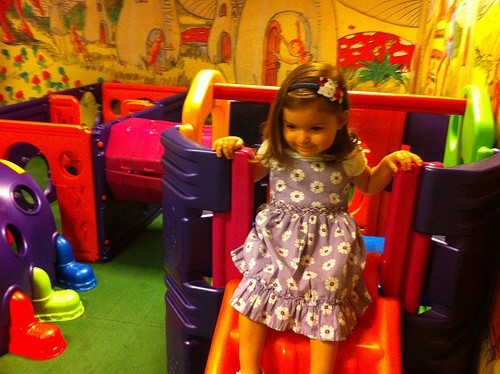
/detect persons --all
[213,61,424,373]
